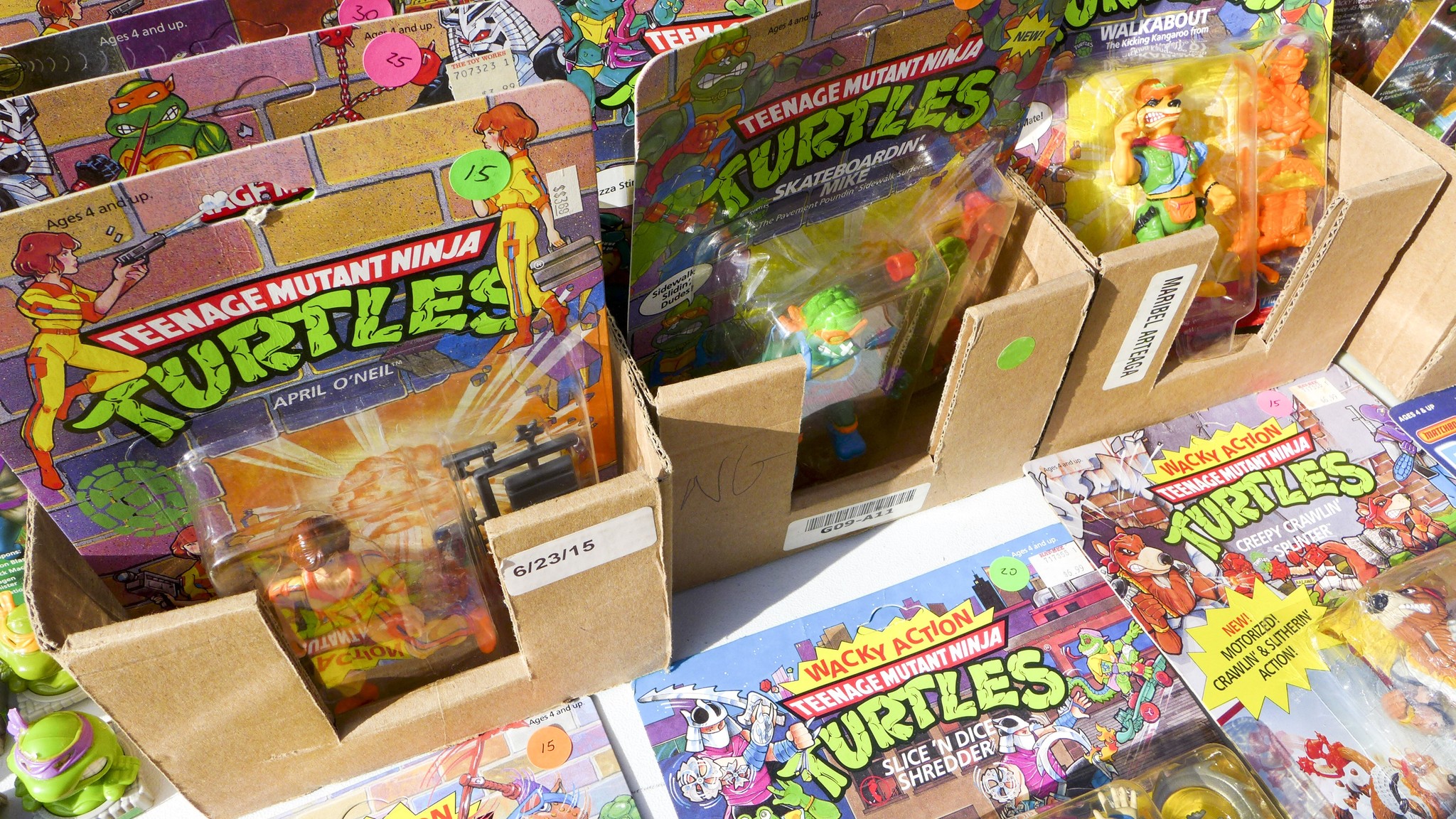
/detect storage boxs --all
[22,73,1456,819]
